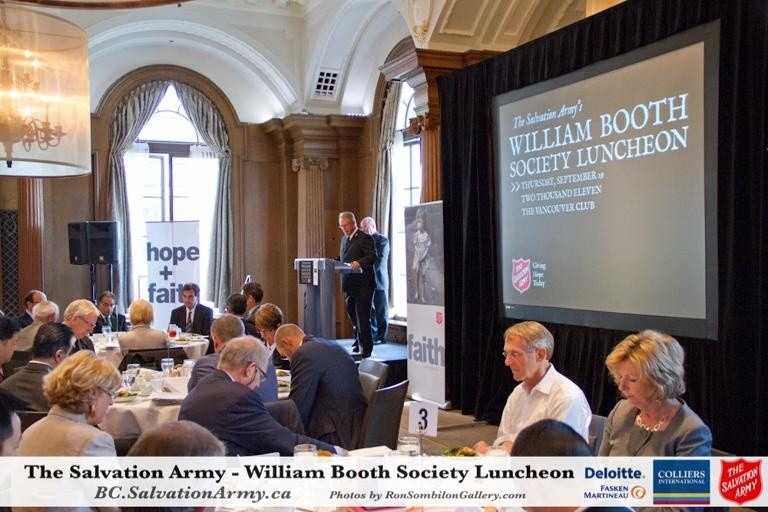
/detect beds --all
[87,332,210,371]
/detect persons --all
[330,212,379,358]
[121,419,225,510]
[360,216,391,344]
[596,328,712,511]
[510,419,636,511]
[0,388,31,511]
[472,320,594,457]
[412,209,431,304]
[10,349,124,511]
[176,335,353,457]
[1,283,365,449]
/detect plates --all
[171,333,206,345]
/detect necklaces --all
[636,405,674,431]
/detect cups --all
[169,324,181,345]
[122,358,194,394]
[483,447,511,456]
[102,325,119,347]
[292,444,319,457]
[397,435,421,456]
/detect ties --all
[186,312,192,331]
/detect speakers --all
[86,220,119,264]
[68,221,85,264]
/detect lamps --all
[0,2,92,178]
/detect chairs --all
[1,347,736,512]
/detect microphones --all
[313,237,336,257]
